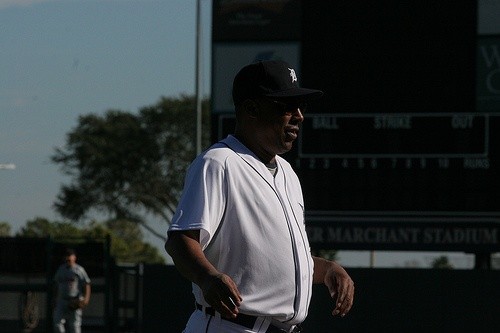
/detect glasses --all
[266,97,309,116]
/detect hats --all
[231,59,324,102]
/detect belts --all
[195,305,299,333]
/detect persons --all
[49,248,91,333]
[167,61,355,333]
